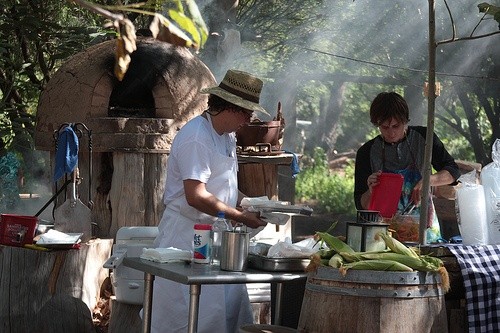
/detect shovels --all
[54,167,92,242]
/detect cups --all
[220,230,252,271]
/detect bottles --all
[210,211,229,266]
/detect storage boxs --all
[0,213,37,248]
[103,226,222,305]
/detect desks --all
[122,256,308,333]
[236,152,304,206]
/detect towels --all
[54,127,80,182]
[286,151,299,174]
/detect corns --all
[308,231,444,272]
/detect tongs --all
[399,199,415,216]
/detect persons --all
[139,70,271,333]
[354,92,462,244]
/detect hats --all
[201,69,273,117]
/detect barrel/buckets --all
[236,121,281,146]
[298,266,448,333]
[0,214,38,247]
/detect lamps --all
[345,210,391,252]
